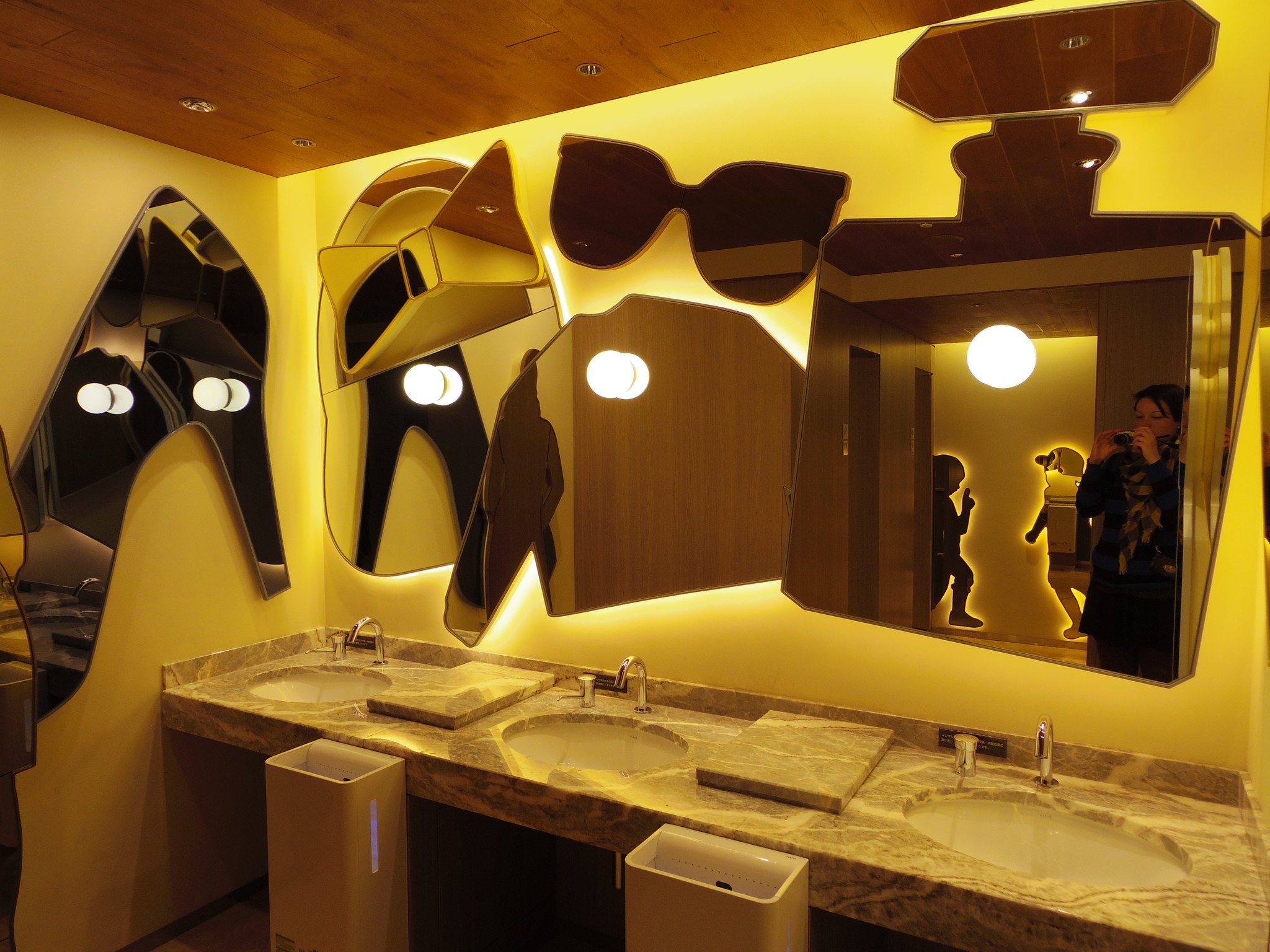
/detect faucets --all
[557,674,598,709]
[346,616,388,665]
[303,633,346,660]
[952,733,978,777]
[1034,714,1059,787]
[614,655,652,714]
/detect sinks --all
[502,712,689,771]
[901,787,1196,888]
[243,665,393,704]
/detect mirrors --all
[318,140,562,577]
[931,453,984,628]
[1024,447,1093,640]
[441,295,806,648]
[0,185,291,951]
[549,134,852,305]
[782,1,1262,690]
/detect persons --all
[1074,382,1186,682]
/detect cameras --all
[1114,431,1135,446]
